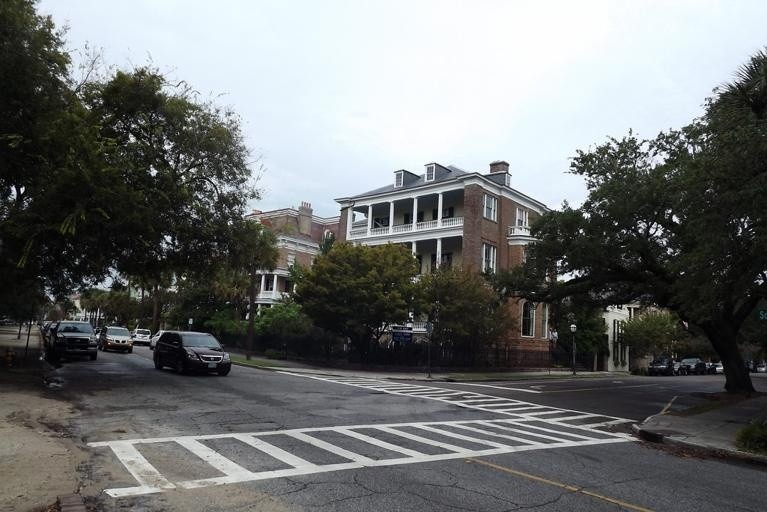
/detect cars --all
[648,358,766,376]
[153,331,231,376]
[41,320,133,360]
[149,329,176,350]
[132,328,152,346]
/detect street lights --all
[570,323,577,374]
